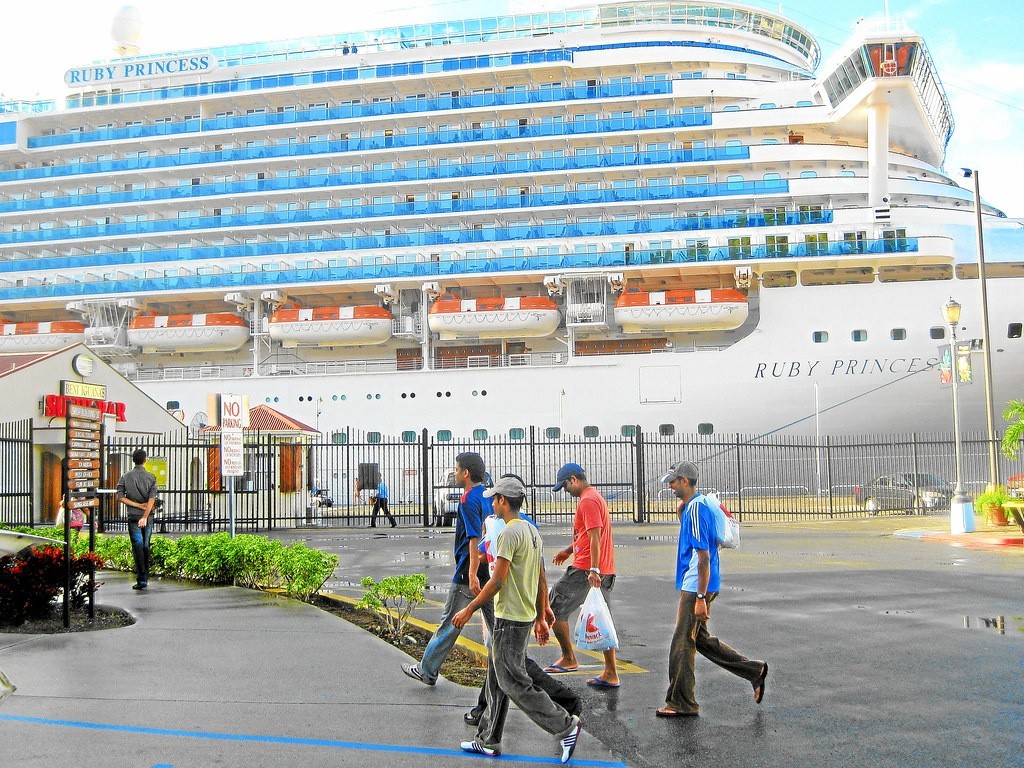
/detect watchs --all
[696,592,706,599]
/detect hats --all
[483,477,526,498]
[661,461,699,483]
[552,463,585,491]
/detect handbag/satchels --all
[574,586,619,651]
[706,492,740,550]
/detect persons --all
[543,464,620,688]
[401,452,495,686]
[340,41,357,56]
[369,477,399,528]
[656,461,768,717]
[354,478,363,505]
[117,450,157,590]
[60,485,90,534]
[452,475,583,764]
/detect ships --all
[0,2,1024,506]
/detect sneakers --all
[751,661,769,703]
[460,740,501,757]
[656,707,698,717]
[559,720,582,763]
[401,663,437,687]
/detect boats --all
[0,322,85,354]
[269,306,394,348]
[127,313,251,354]
[614,283,749,334]
[429,296,561,341]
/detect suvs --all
[434,469,496,526]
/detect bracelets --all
[589,567,600,575]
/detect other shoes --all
[463,710,479,725]
[391,524,397,528]
[132,581,147,590]
[370,526,376,527]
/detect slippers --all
[587,677,620,687]
[543,664,578,673]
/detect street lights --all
[941,295,975,504]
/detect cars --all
[855,471,958,515]
